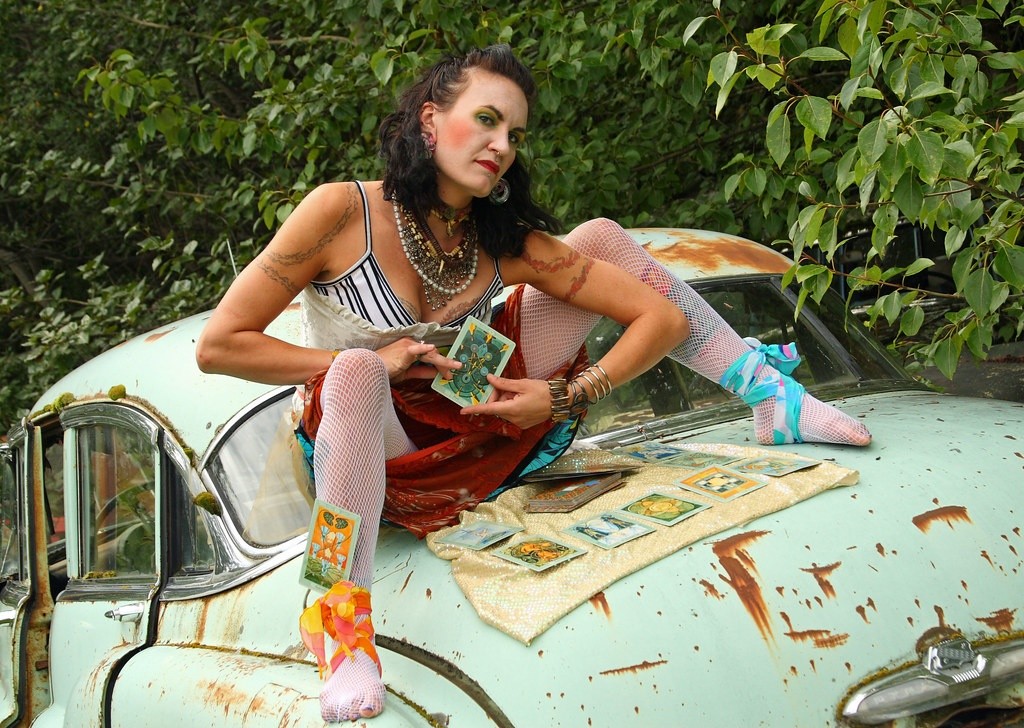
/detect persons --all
[193,42,874,725]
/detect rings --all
[416,340,426,359]
[495,414,499,419]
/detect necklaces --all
[391,187,479,313]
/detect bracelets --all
[548,363,613,422]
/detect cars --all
[3,228,1024,726]
